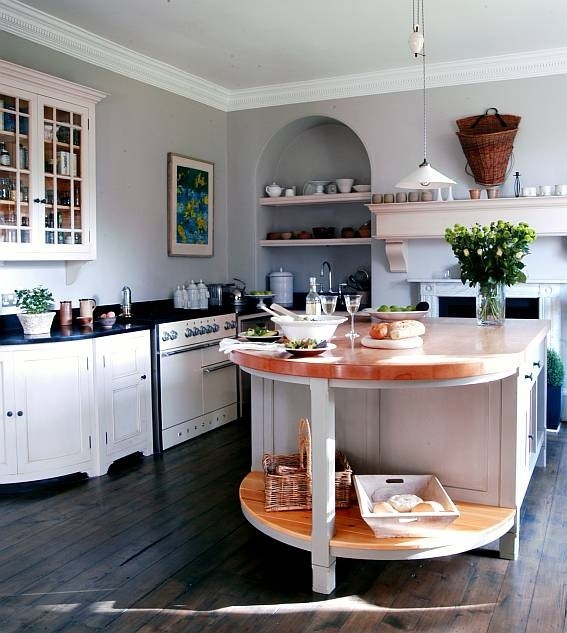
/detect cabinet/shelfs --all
[95,328,152,459]
[1,342,92,490]
[156,311,242,452]
[519,340,547,494]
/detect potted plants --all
[14,284,56,339]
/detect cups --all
[60,301,73,324]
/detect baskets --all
[263,416,352,512]
[456,108,521,132]
[456,129,517,184]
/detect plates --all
[352,184,371,193]
[282,344,335,355]
[237,331,284,343]
[364,305,429,321]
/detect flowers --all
[444,219,537,283]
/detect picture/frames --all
[165,152,214,258]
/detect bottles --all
[306,276,321,315]
[1,142,11,165]
[180,284,188,309]
[186,278,200,310]
[1,177,11,199]
[1,213,65,244]
[171,285,183,307]
[197,279,210,309]
[60,188,79,206]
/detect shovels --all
[271,302,312,321]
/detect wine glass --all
[322,295,338,314]
[344,293,362,339]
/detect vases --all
[475,279,506,326]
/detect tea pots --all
[226,285,245,306]
[76,298,97,323]
[264,179,284,197]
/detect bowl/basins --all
[337,178,354,192]
[269,313,350,343]
[98,318,117,329]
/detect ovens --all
[159,340,245,430]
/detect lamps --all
[392,158,460,189]
[407,25,426,64]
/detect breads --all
[369,322,389,339]
[389,320,426,339]
[372,494,445,514]
